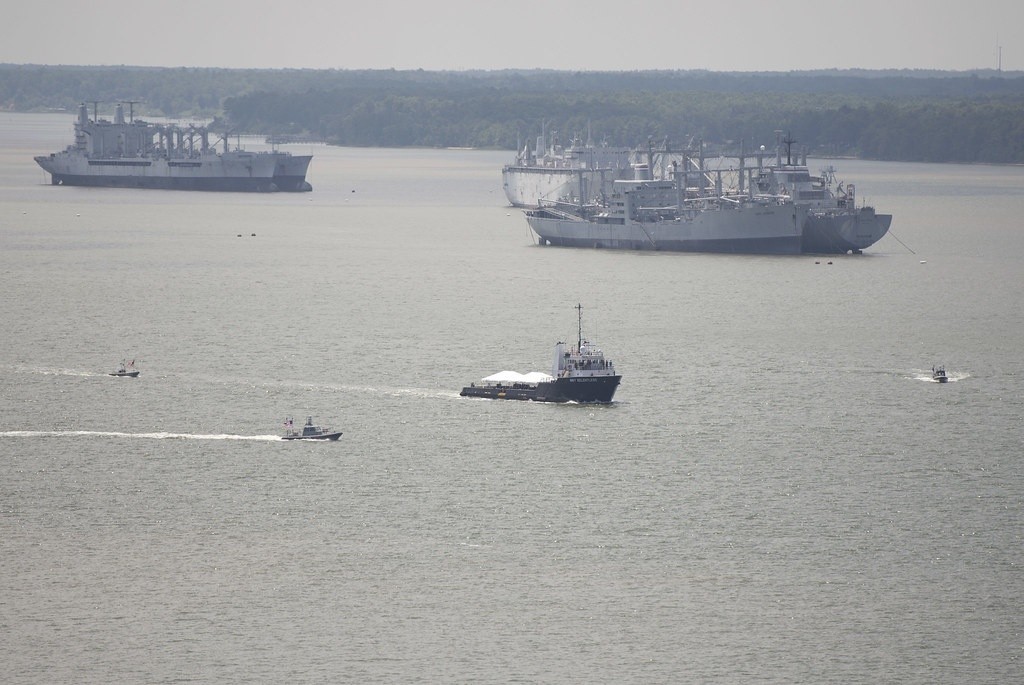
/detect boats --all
[280,413,345,441]
[932,364,949,383]
[461,302,623,402]
[108,359,141,377]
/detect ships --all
[31,97,315,188]
[500,116,894,253]
